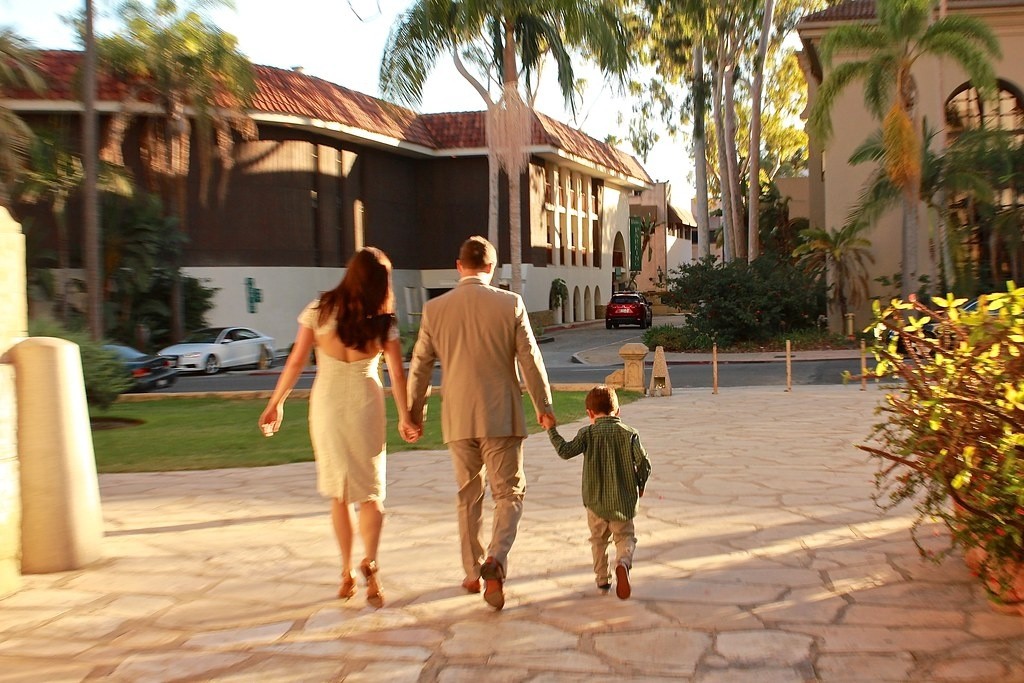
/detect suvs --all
[605,291,653,329]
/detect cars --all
[102,342,178,393]
[153,326,275,374]
[885,298,1024,357]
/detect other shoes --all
[463,579,482,593]
[481,556,505,611]
[615,560,632,600]
[596,581,613,594]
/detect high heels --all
[339,568,358,600]
[360,558,385,610]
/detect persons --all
[405,237,556,610]
[259,246,420,608]
[543,385,652,599]
